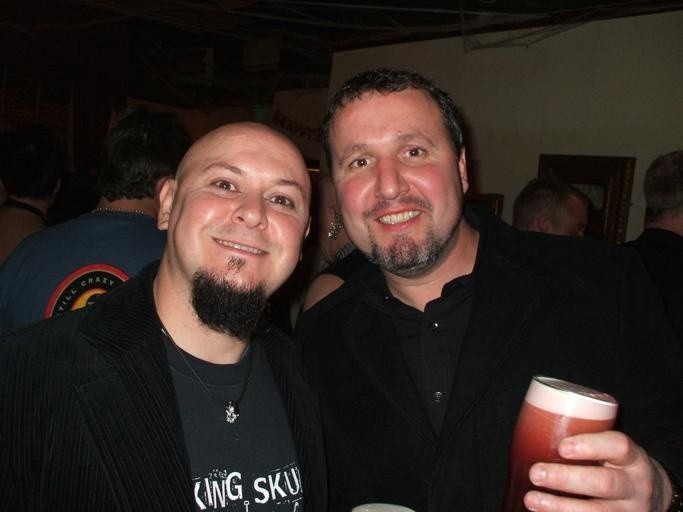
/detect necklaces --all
[91,205,145,219]
[157,308,256,429]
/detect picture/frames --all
[536,154,636,243]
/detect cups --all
[508,372,617,512]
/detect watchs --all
[667,480,681,512]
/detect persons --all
[507,167,588,242]
[300,63,679,512]
[299,164,377,313]
[474,207,601,324]
[0,107,194,339]
[0,119,325,509]
[591,144,682,457]
[0,135,63,266]
